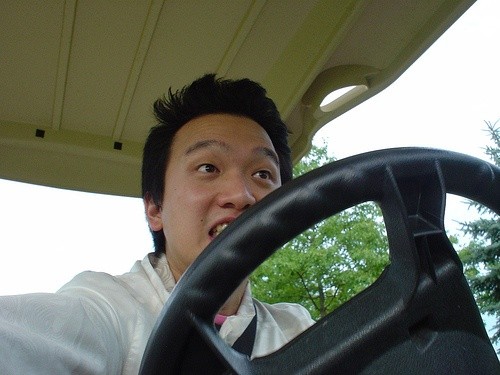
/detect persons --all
[2,70,317,374]
